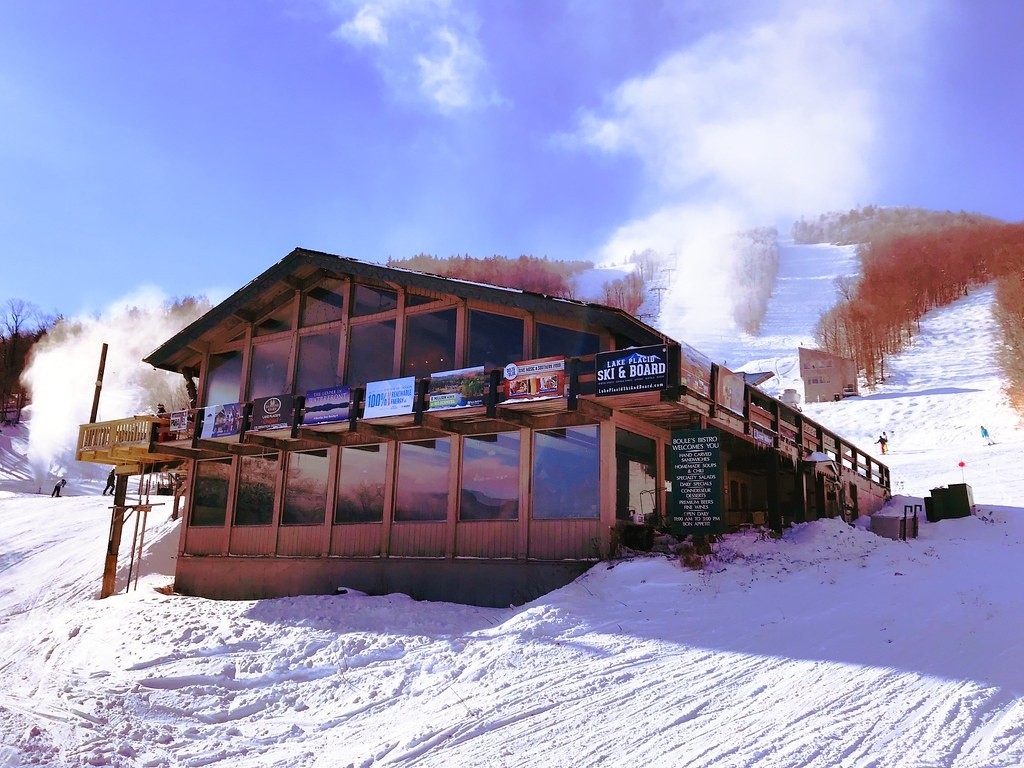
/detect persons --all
[157,404,166,413]
[980,426,995,445]
[102,469,115,496]
[51,479,66,497]
[875,432,889,455]
